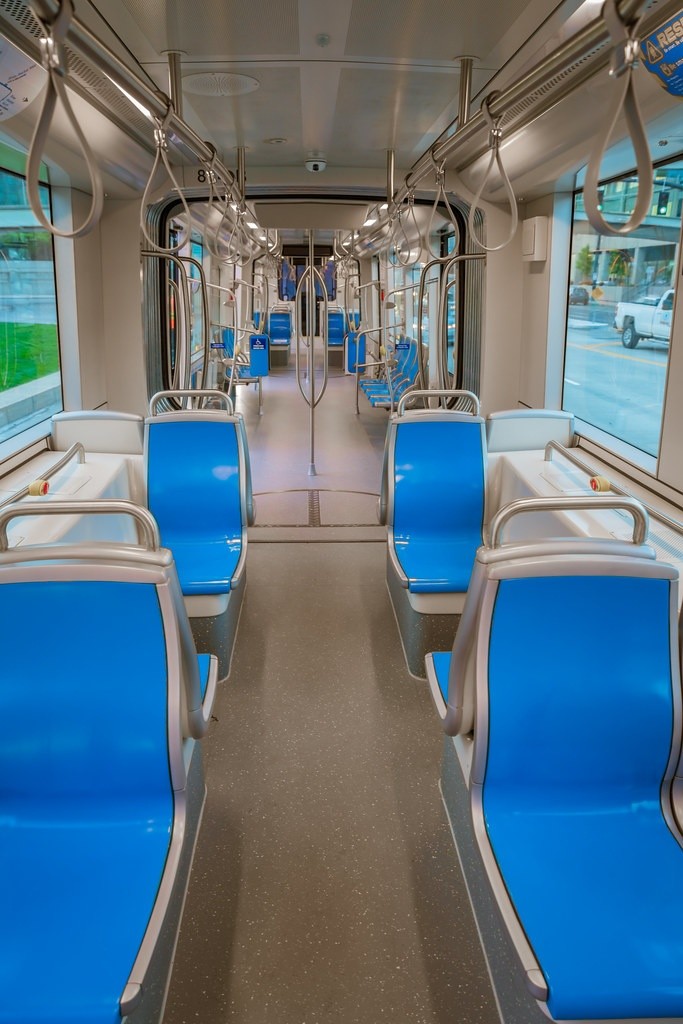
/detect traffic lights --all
[657,191,670,216]
[597,190,604,213]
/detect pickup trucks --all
[612,289,676,348]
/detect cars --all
[414,292,454,346]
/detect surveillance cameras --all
[306,161,326,172]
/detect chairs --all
[0,299,683,1024]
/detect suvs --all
[570,287,588,305]
